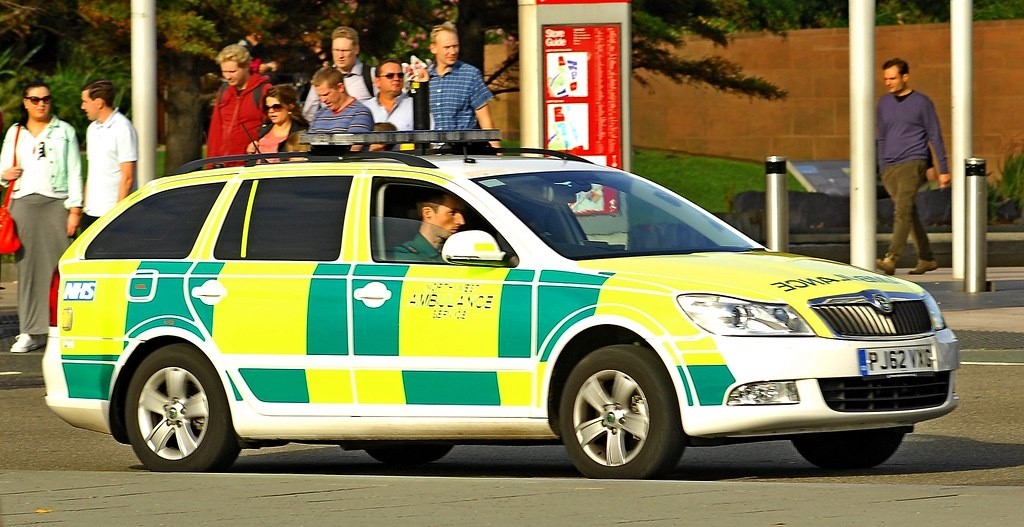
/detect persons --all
[0,82,84,352]
[205,45,273,170]
[246,86,309,165]
[392,188,465,262]
[876,58,951,276]
[80,77,138,233]
[425,22,503,156]
[303,25,435,151]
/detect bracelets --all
[70,207,82,215]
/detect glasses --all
[262,104,286,114]
[24,94,53,105]
[376,72,404,79]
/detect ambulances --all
[41,121,961,483]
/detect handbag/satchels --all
[0,205,22,255]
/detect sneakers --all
[10,332,48,352]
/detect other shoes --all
[908,259,937,275]
[876,258,896,275]
